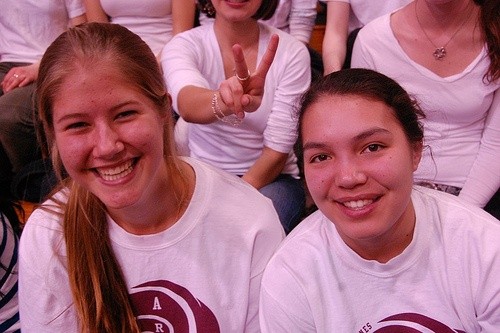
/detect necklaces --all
[415,1,475,60]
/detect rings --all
[236,69,250,81]
[15,74,20,78]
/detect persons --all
[0,0,500,333]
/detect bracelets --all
[212,90,244,127]
